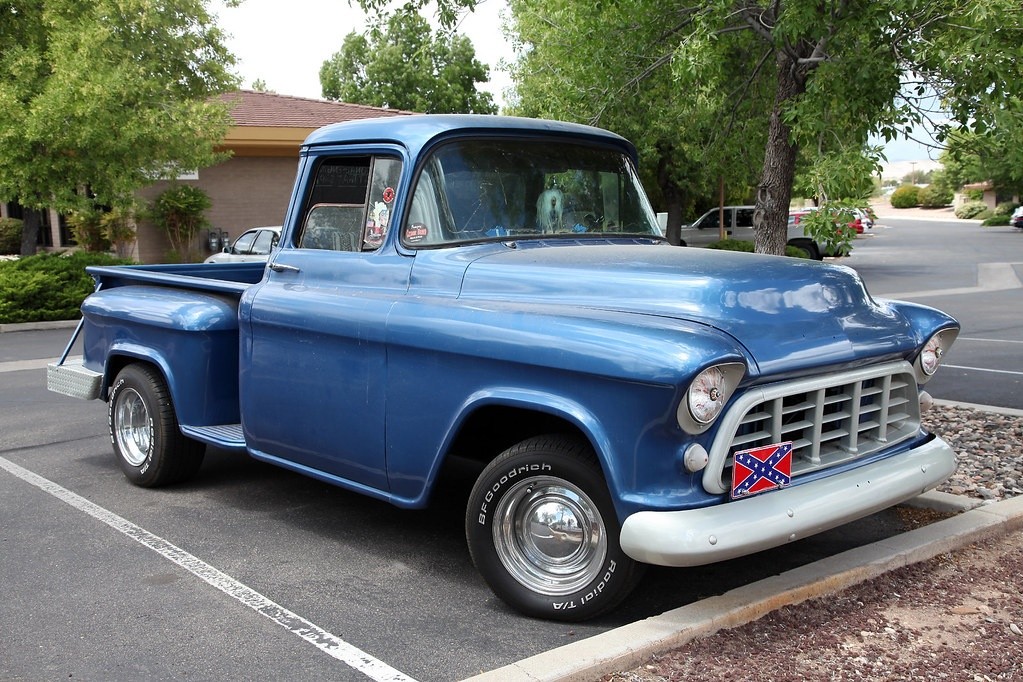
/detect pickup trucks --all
[677,206,844,262]
[46,113,959,623]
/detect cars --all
[202,224,285,264]
[789,206,875,236]
[1009,205,1023,229]
